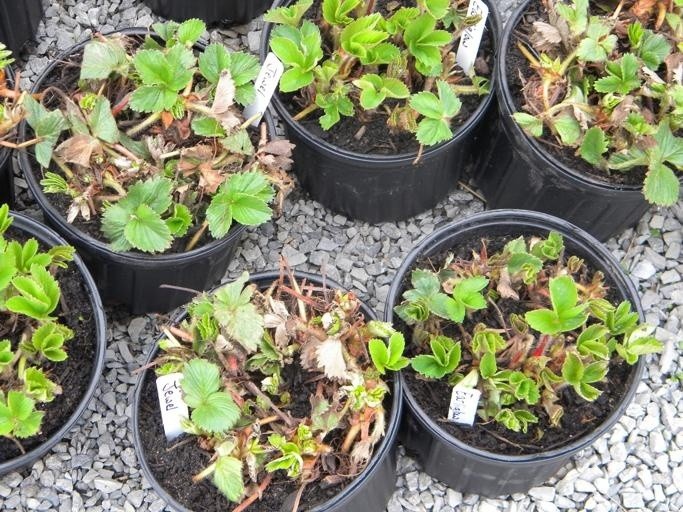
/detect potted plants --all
[0,209,107,477]
[385,208,648,499]
[18,18,296,316]
[472,1,683,242]
[130,258,404,512]
[258,1,503,223]
